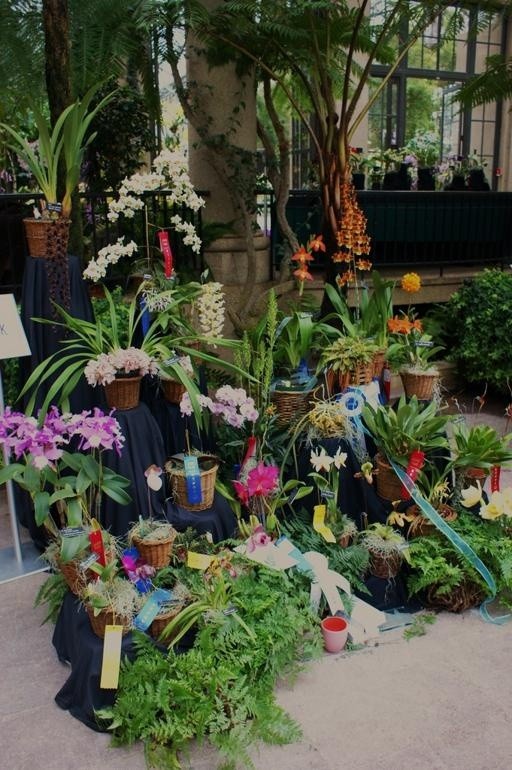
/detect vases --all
[235,531,278,546]
[60,534,119,595]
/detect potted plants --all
[141,573,190,645]
[131,517,178,572]
[363,525,405,579]
[78,577,138,637]
[0,76,124,261]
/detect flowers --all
[0,144,512,535]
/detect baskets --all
[159,373,194,405]
[129,524,178,572]
[372,452,420,503]
[53,538,116,597]
[397,366,439,402]
[24,217,74,258]
[334,501,459,581]
[83,600,133,641]
[269,383,322,428]
[137,598,185,647]
[163,455,219,514]
[101,375,142,411]
